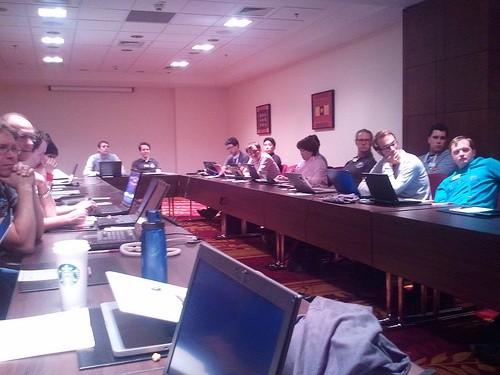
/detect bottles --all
[141,210,167,284]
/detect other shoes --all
[197,209,212,220]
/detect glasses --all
[378,140,399,151]
[248,150,259,155]
[357,139,371,145]
[18,133,36,142]
[0,144,21,154]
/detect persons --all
[260,134,330,244]
[131,143,162,174]
[0,112,99,321]
[417,124,459,175]
[83,141,126,176]
[262,137,281,173]
[364,135,500,303]
[294,129,432,297]
[264,129,377,272]
[216,142,281,235]
[196,137,248,220]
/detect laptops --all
[97,161,122,177]
[213,164,236,178]
[228,163,253,179]
[79,180,170,249]
[325,169,370,198]
[361,171,421,205]
[100,270,187,358]
[71,163,78,177]
[203,162,217,172]
[159,240,302,375]
[97,177,161,229]
[283,173,337,193]
[98,168,143,215]
[259,170,289,184]
[243,164,261,181]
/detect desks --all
[0,171,500,375]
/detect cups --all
[52,239,91,311]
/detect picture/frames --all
[311,89,334,128]
[256,102,271,134]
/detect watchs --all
[37,186,51,198]
